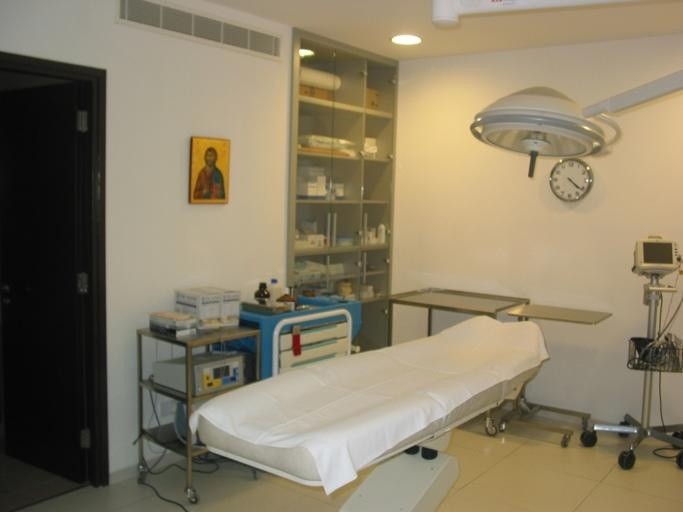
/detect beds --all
[189,316,551,495]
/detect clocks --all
[549,158,593,202]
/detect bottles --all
[377,223,385,243]
[254,282,270,305]
[268,279,282,302]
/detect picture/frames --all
[189,137,231,204]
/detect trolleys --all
[383,285,531,435]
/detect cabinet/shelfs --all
[287,26,399,356]
[213,292,363,381]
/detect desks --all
[387,289,530,346]
